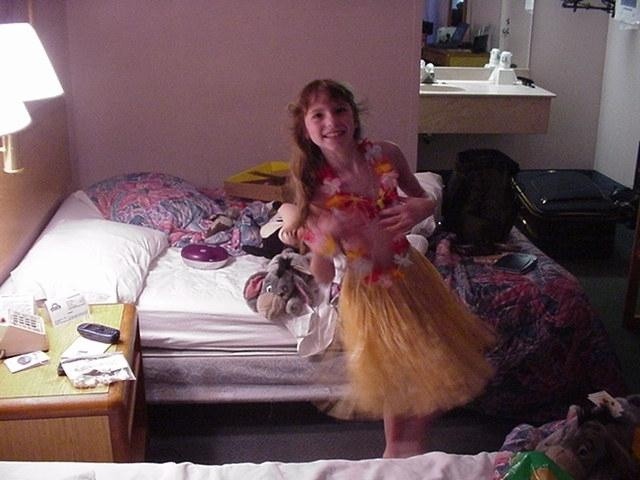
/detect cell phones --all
[77,323,120,343]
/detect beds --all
[1,189,631,422]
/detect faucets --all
[421,62,436,83]
[420,58,426,70]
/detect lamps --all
[0,22,66,173]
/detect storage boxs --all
[224,161,295,202]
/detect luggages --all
[509,169,633,256]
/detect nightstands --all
[1,303,147,461]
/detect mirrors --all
[421,0,536,71]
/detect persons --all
[287,79,499,458]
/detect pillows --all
[83,173,223,246]
[1,190,170,303]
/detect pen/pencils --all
[478,26,485,36]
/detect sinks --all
[420,85,465,92]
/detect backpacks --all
[442,148,520,242]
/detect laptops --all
[429,22,469,49]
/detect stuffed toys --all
[244,248,320,322]
[494,390,640,480]
[242,203,299,260]
